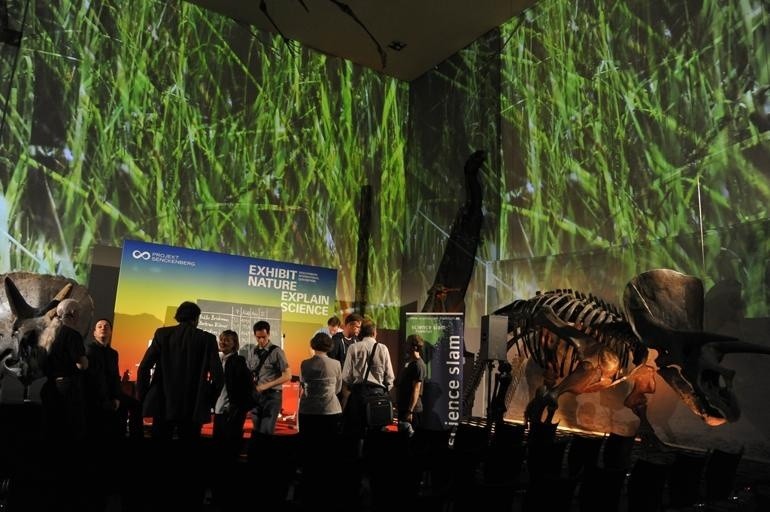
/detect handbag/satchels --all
[343,382,394,429]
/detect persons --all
[213,329,260,462]
[85,319,124,432]
[246,319,291,437]
[35,299,61,413]
[297,312,427,444]
[38,297,94,415]
[136,301,225,439]
